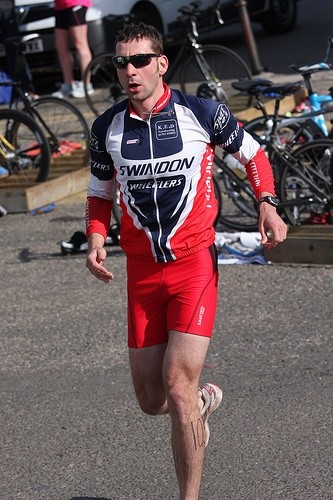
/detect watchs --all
[258,196,279,207]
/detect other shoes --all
[51,83,72,99]
[69,80,94,97]
[197,382,223,447]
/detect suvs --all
[0,0,297,85]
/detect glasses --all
[112,54,161,69]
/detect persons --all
[52,0,94,100]
[85,24,288,500]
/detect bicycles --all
[197,63,333,232]
[0,33,91,184]
[84,0,252,118]
